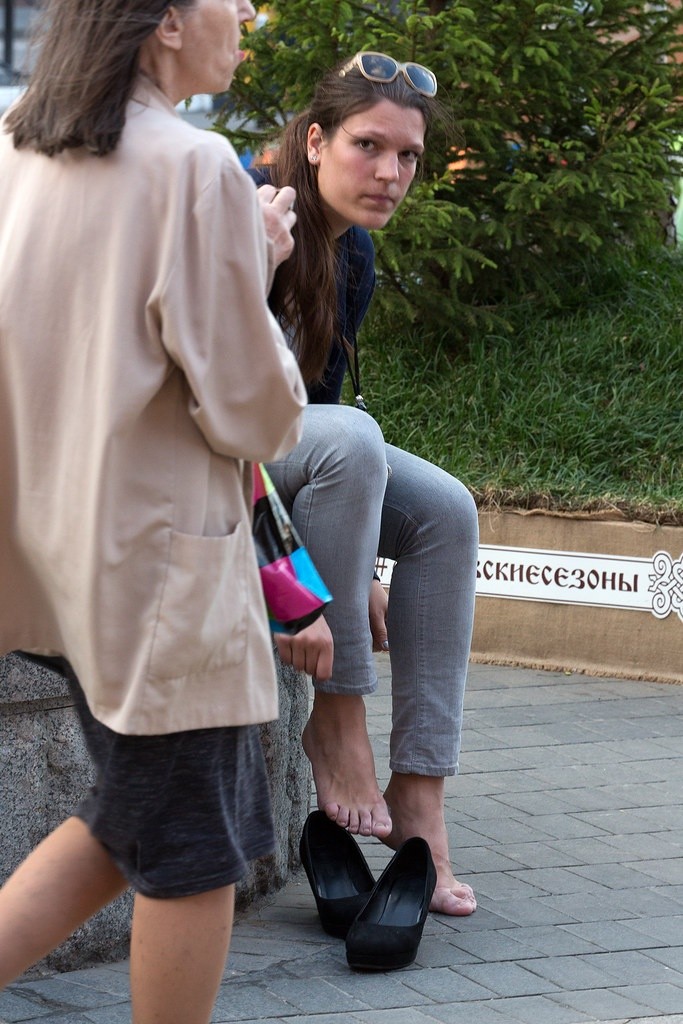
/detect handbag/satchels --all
[252,461,335,637]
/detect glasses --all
[338,51,437,98]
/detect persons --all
[0,0,309,1024]
[245,50,479,916]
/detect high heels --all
[299,810,375,939]
[345,837,437,972]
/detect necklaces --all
[335,232,367,412]
